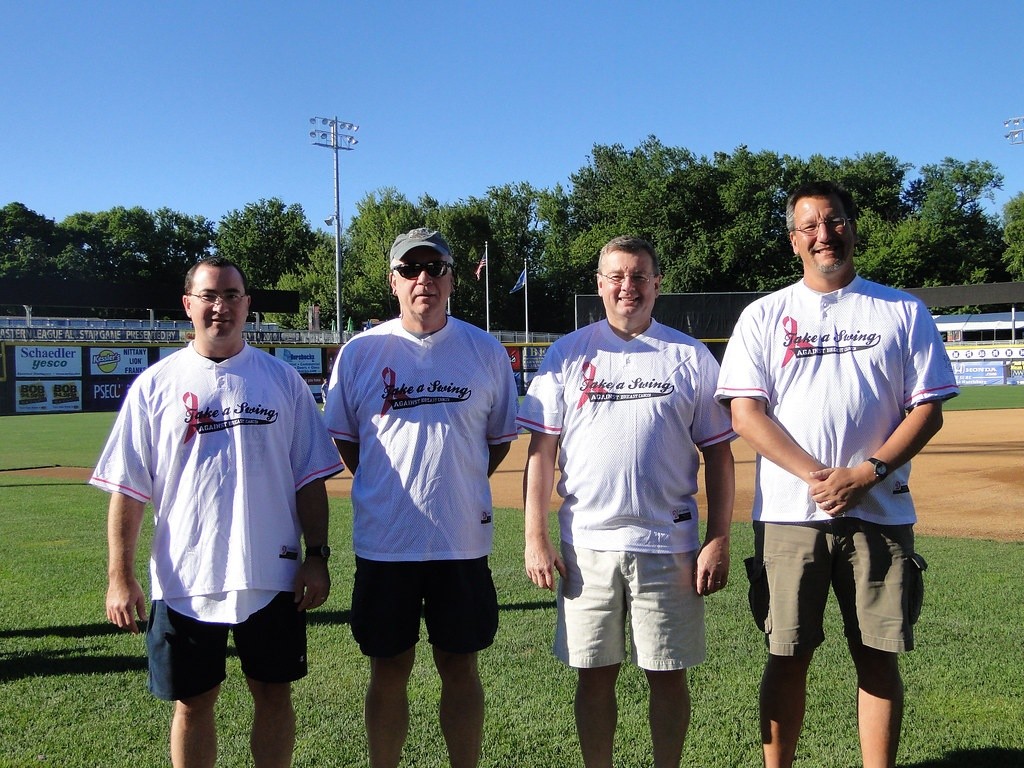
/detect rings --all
[320,596,327,601]
[824,501,832,509]
[715,581,721,583]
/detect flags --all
[510,269,526,293]
[474,252,486,281]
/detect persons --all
[324,229,521,768]
[514,235,740,768]
[713,181,958,768]
[87,259,346,768]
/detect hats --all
[390,228,449,263]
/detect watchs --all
[305,545,330,558]
[868,458,887,480]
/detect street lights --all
[306,115,358,345]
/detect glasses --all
[390,261,453,280]
[598,272,656,286]
[187,292,246,304]
[788,217,852,236]
